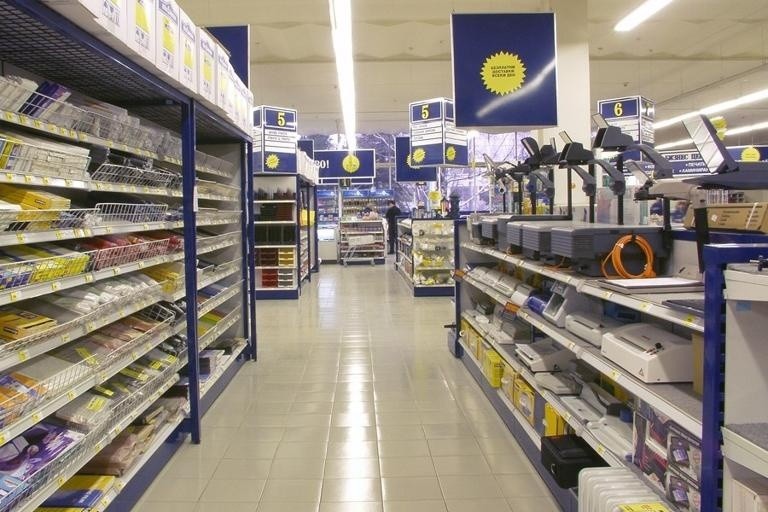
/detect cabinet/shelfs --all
[318,225,337,265]
[0,0,259,512]
[393,215,454,298]
[446,193,768,512]
[253,105,320,301]
[316,189,340,225]
[337,220,387,267]
[340,189,395,220]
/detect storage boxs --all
[682,200,768,235]
[0,0,254,137]
[458,318,612,490]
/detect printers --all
[514,336,577,373]
[494,274,525,298]
[564,310,625,349]
[466,265,491,282]
[479,268,506,289]
[600,322,693,383]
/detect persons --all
[385,200,401,254]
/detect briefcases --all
[541,434,609,488]
